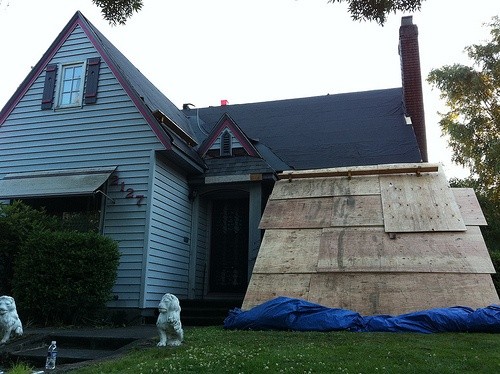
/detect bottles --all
[45,340,57,370]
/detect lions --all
[156,293,184,347]
[0,295,23,344]
